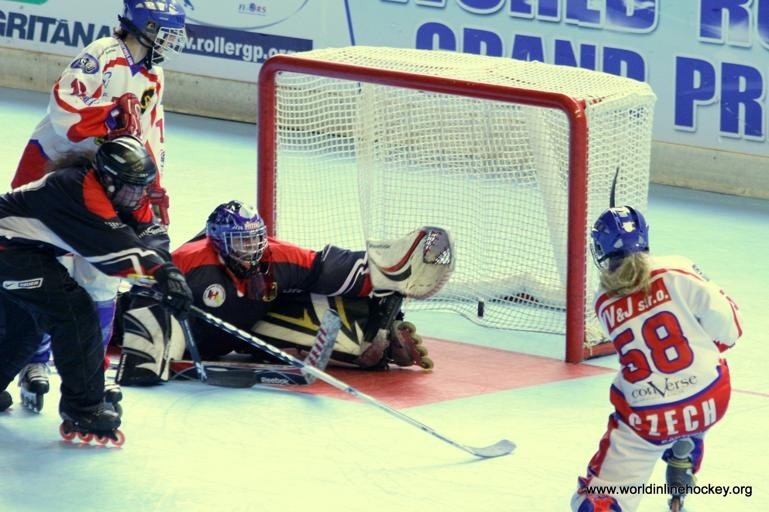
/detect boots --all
[665,437,696,497]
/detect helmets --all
[204,200,270,278]
[118,0,187,70]
[589,203,649,271]
[92,136,157,211]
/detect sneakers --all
[19,363,49,394]
[390,320,413,366]
[59,381,123,431]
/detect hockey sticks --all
[108,313,341,385]
[187,307,515,459]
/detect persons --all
[170,201,453,373]
[572,206,743,512]
[13,1,188,398]
[1,135,158,432]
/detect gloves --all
[152,261,193,320]
[106,93,141,138]
